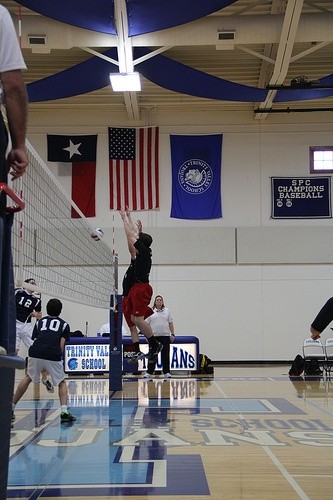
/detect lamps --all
[110,72,140,92]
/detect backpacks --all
[304,356,323,375]
[288,354,306,376]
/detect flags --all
[169,134,223,219]
[46,134,98,219]
[107,126,160,210]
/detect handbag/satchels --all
[191,354,214,374]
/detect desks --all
[63,336,199,377]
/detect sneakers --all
[165,373,172,378]
[142,372,154,377]
[11,414,15,423]
[42,377,54,393]
[146,342,163,359]
[128,351,145,364]
[60,411,76,422]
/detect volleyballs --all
[90,228,103,241]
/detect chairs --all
[302,336,333,381]
[99,323,127,336]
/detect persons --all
[142,296,175,379]
[13,278,54,392]
[97,322,127,336]
[310,297,333,340]
[10,298,77,423]
[0,3,29,225]
[120,205,163,363]
